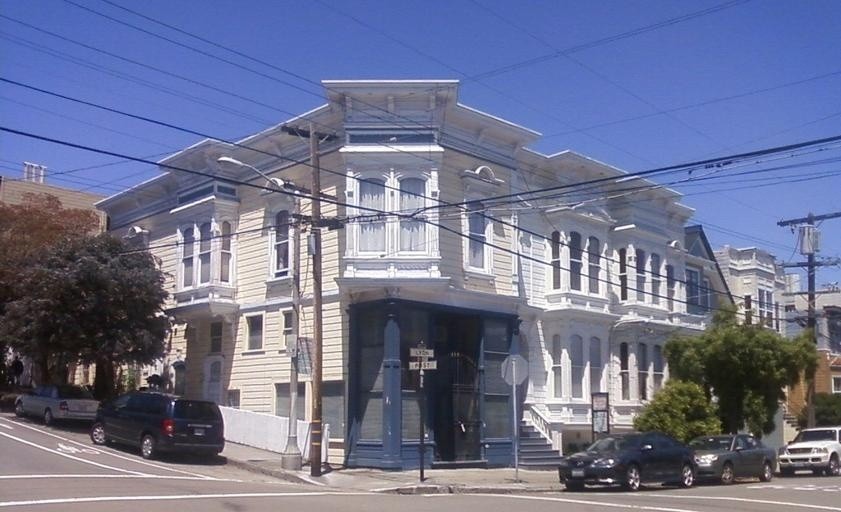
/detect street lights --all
[214,153,302,471]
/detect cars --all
[557,431,695,492]
[683,432,777,485]
[14,382,98,427]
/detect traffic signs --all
[407,358,437,370]
[408,347,433,358]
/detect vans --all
[90,390,226,461]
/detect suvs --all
[777,426,841,477]
